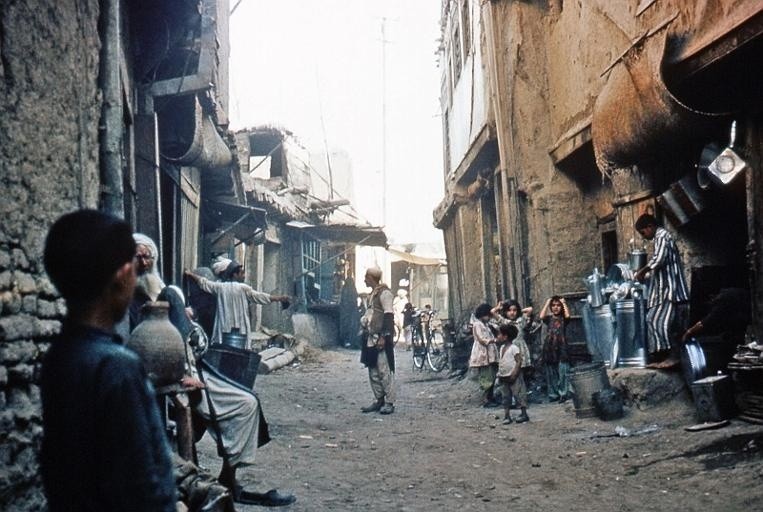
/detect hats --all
[222,260,242,278]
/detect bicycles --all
[357,299,401,348]
[408,310,448,372]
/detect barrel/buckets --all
[564,360,613,420]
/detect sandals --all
[360,401,386,412]
[379,401,395,414]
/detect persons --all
[634,214,689,368]
[490,300,533,368]
[360,271,395,414]
[426,305,431,311]
[129,233,259,502]
[402,303,415,351]
[41,210,176,512]
[540,296,570,403]
[469,305,500,406]
[212,256,232,282]
[682,283,750,374]
[497,325,529,424]
[184,233,290,350]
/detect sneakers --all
[234,481,297,507]
[515,415,530,423]
[502,417,511,425]
